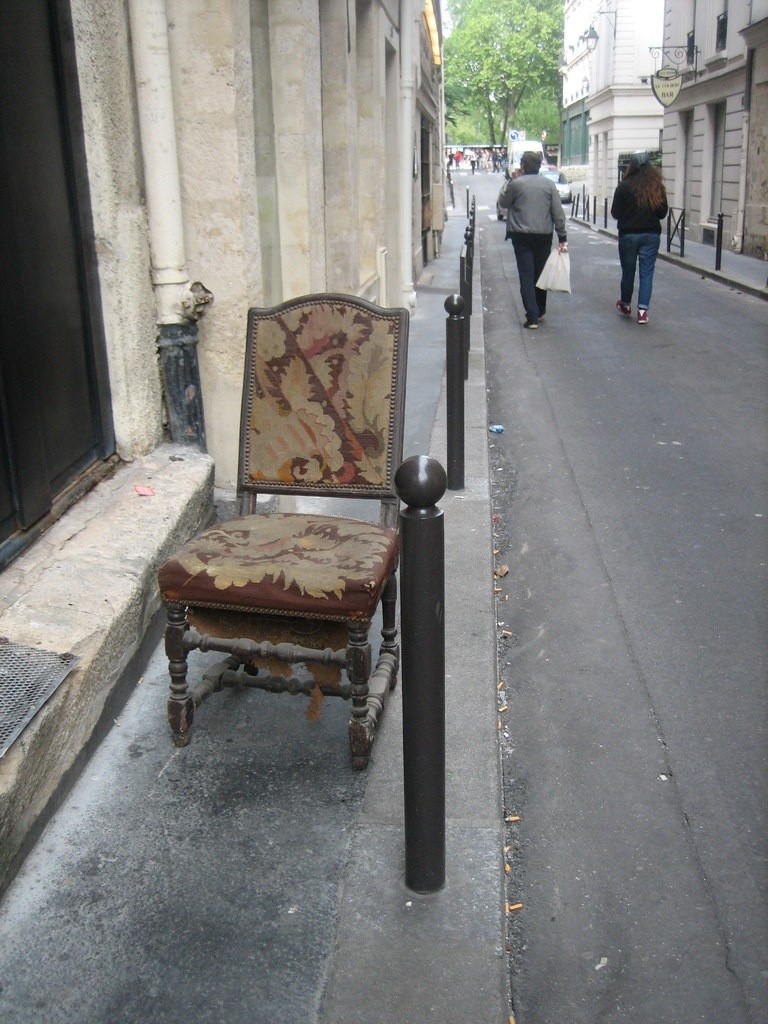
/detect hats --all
[630,152,649,169]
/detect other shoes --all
[524,321,538,329]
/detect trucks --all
[505,140,550,180]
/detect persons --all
[610,151,668,322]
[499,150,567,328]
[449,148,507,175]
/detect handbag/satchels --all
[535,242,572,294]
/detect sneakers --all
[636,310,649,323]
[617,300,632,316]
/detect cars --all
[539,169,573,204]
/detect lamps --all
[582,12,616,55]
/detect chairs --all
[158,293,411,772]
